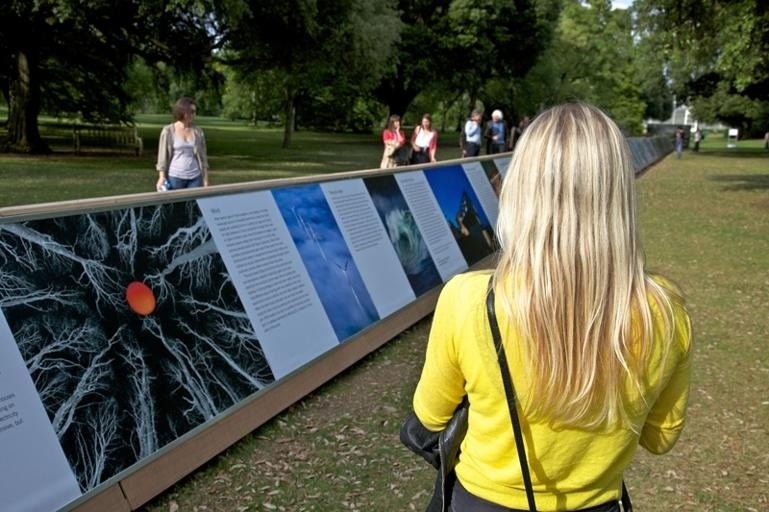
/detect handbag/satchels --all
[398,400,468,476]
[407,146,413,159]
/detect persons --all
[671,126,685,160]
[692,128,703,153]
[408,103,695,511]
[483,109,508,154]
[153,95,212,192]
[408,114,438,164]
[379,115,406,169]
[508,115,531,152]
[461,108,483,159]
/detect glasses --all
[190,109,197,114]
